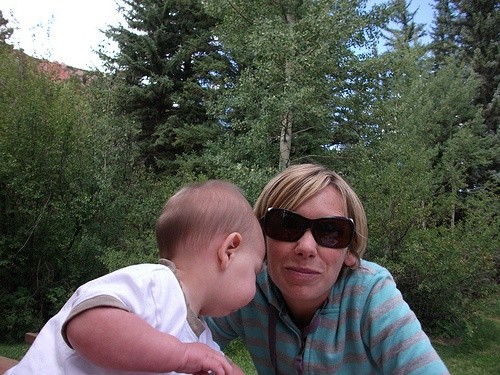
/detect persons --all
[198,164,451,375]
[4,179,266,375]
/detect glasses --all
[258,207,355,249]
